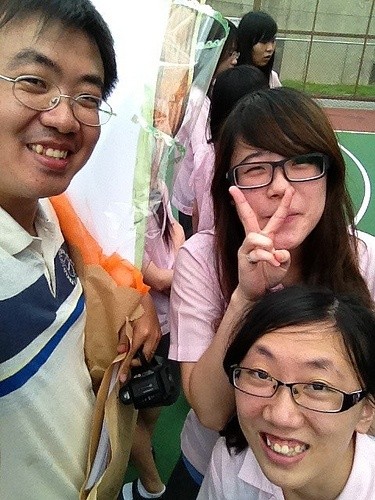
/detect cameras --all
[118,361,180,408]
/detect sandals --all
[117,477,167,500]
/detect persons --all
[163,86,375,499]
[0,1,162,499]
[119,10,283,500]
[195,282,375,500]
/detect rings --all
[247,253,253,263]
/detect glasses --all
[1,74,117,128]
[231,366,370,414]
[222,50,240,60]
[223,152,329,190]
